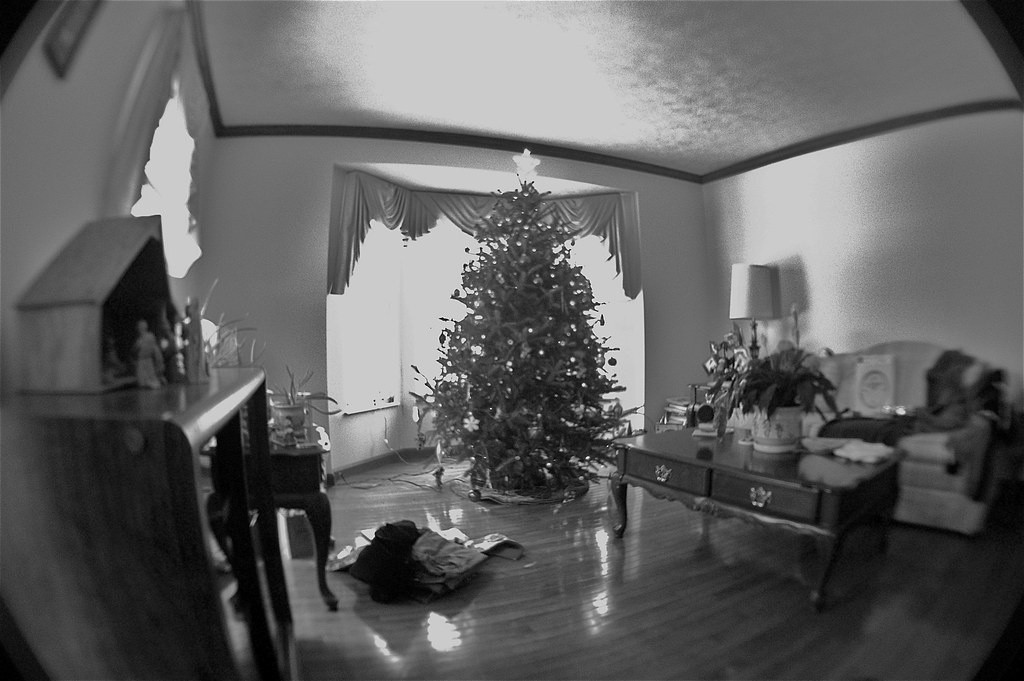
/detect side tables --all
[687,383,726,429]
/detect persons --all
[132,320,165,389]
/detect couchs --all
[729,340,1012,535]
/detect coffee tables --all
[610,428,908,613]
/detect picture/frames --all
[45,0,102,77]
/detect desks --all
[200,440,264,612]
[270,422,339,612]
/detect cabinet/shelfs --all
[1,365,299,681]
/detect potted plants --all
[705,341,839,445]
[267,363,342,428]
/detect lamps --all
[729,263,774,360]
